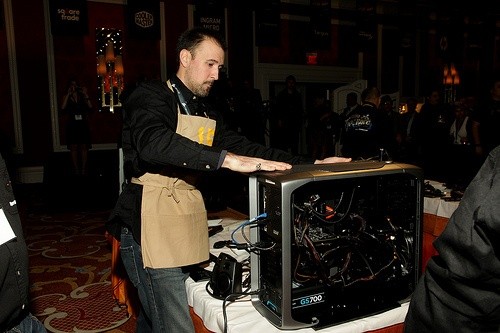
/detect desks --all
[104,206,413,333]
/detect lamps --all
[442,63,461,105]
[95,24,127,114]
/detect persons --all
[412,90,440,164]
[444,102,482,172]
[60,77,97,175]
[276,73,304,159]
[404,142,499,333]
[102,29,353,330]
[371,95,397,160]
[397,99,419,163]
[339,92,369,156]
[309,101,340,160]
[1,156,49,333]
[238,86,268,149]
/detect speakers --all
[213,260,242,301]
[210,252,237,289]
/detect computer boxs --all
[249,161,425,330]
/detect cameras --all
[75,86,82,92]
